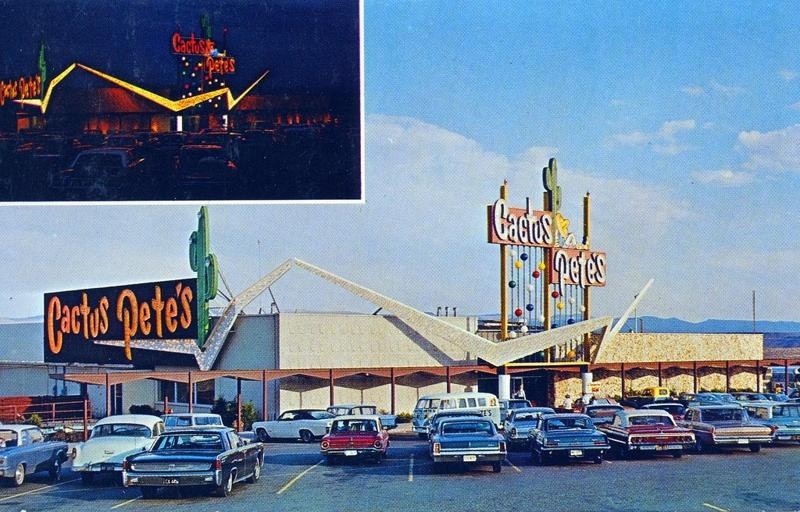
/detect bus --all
[768,365,800,390]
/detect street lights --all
[639,318,643,333]
[634,293,641,334]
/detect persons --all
[514,385,525,399]
[774,383,781,394]
[563,394,574,413]
[581,391,589,414]
